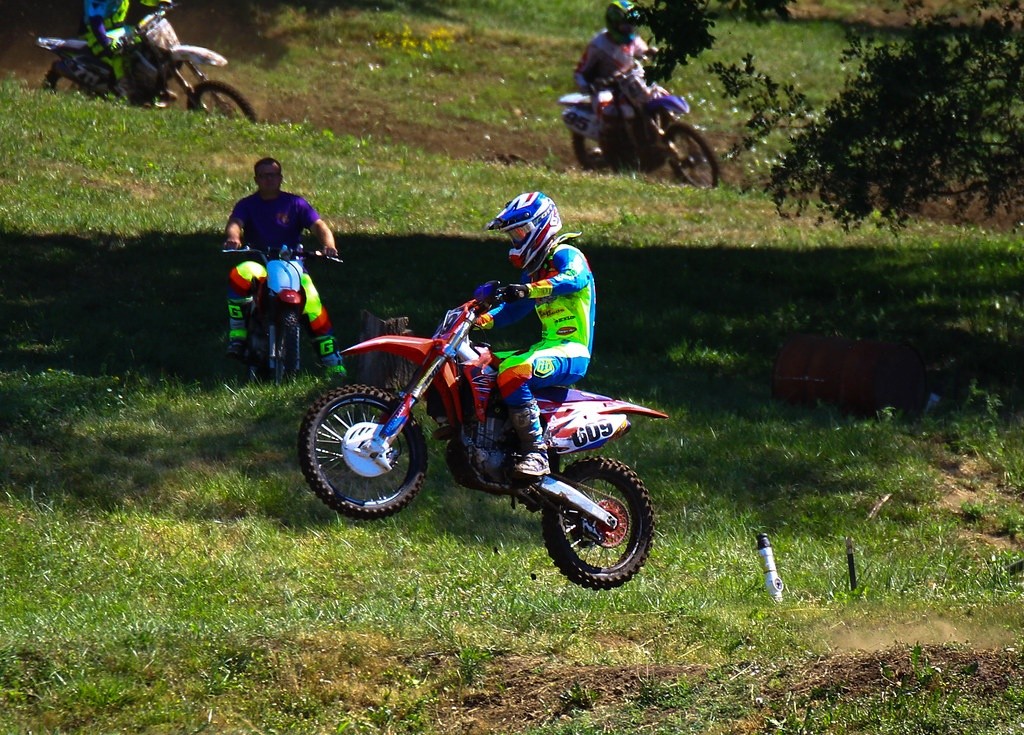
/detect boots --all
[226,295,256,340]
[312,335,348,384]
[506,398,551,475]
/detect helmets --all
[605,0,641,43]
[486,192,563,271]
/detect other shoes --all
[156,89,178,101]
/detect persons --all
[574,0,659,155]
[84,0,178,103]
[223,157,349,381]
[471,192,596,475]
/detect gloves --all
[104,39,123,59]
[495,282,528,303]
[587,76,608,95]
[158,0,173,10]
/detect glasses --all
[256,172,281,180]
[508,202,555,245]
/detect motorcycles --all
[558,48,722,192]
[222,243,344,387]
[32,1,259,127]
[296,278,655,590]
